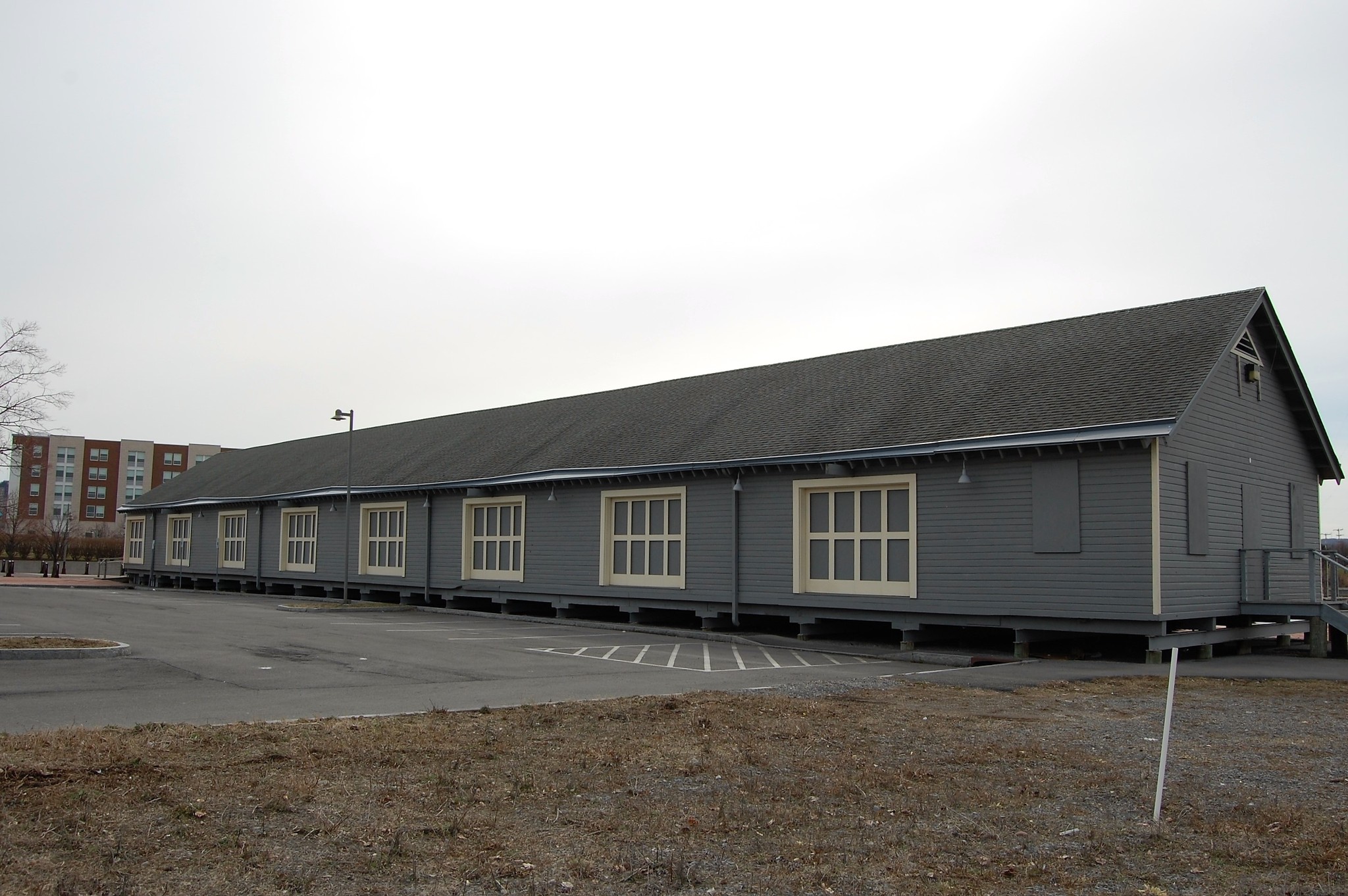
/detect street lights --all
[330,408,353,605]
[61,503,74,575]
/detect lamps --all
[197,508,205,518]
[955,457,973,485]
[422,494,432,509]
[255,505,262,515]
[732,471,744,493]
[149,511,156,521]
[546,485,559,502]
[328,499,338,513]
